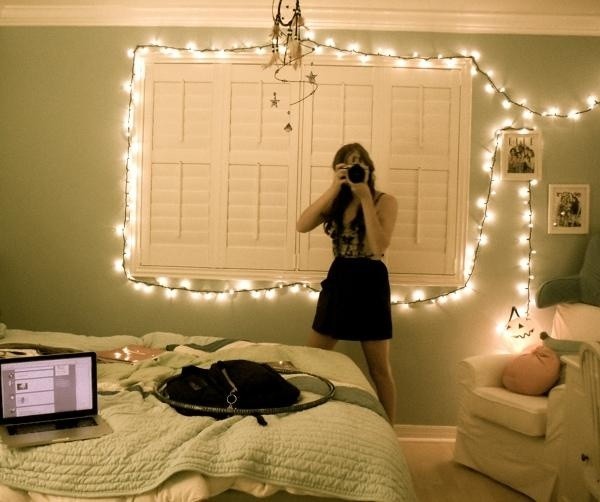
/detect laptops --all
[0,352,114,450]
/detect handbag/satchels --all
[166,360,299,420]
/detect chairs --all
[452,303,599,501]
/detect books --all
[100,344,165,364]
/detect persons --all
[296,143,399,431]
[507,140,535,172]
[553,190,582,226]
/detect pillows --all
[503,331,594,397]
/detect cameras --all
[341,165,369,183]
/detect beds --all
[0,322,417,501]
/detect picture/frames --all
[502,129,542,181]
[546,183,591,236]
